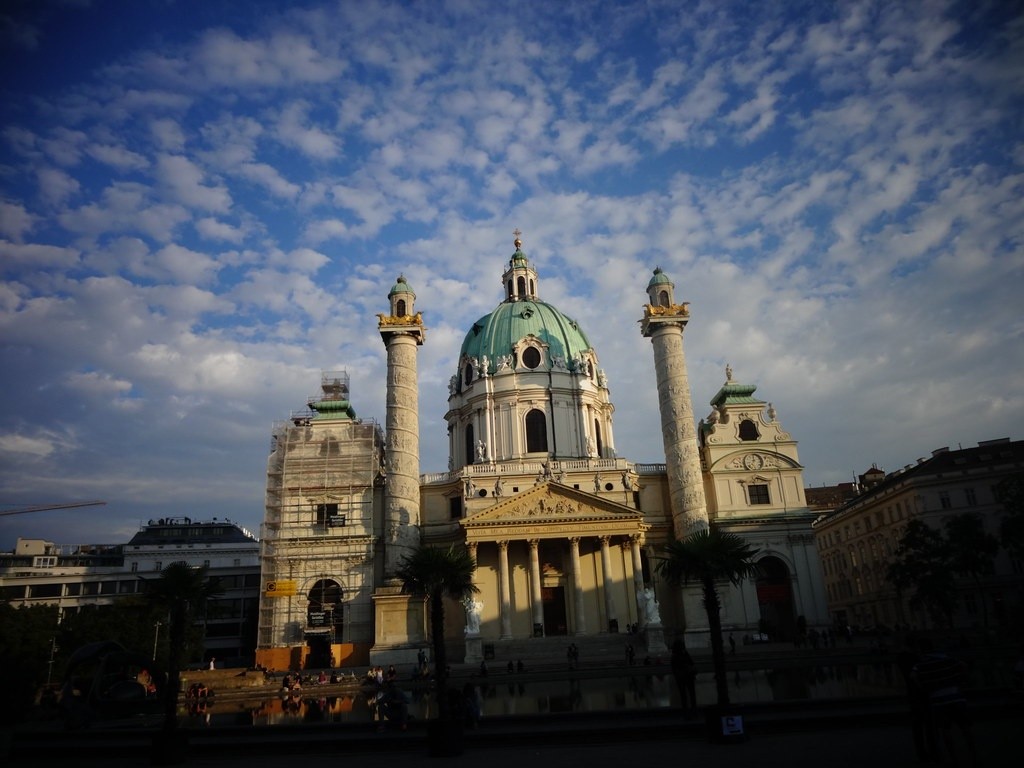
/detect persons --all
[672,640,700,721]
[728,633,737,656]
[757,614,986,768]
[186,676,670,724]
[178,610,664,697]
[376,683,410,732]
